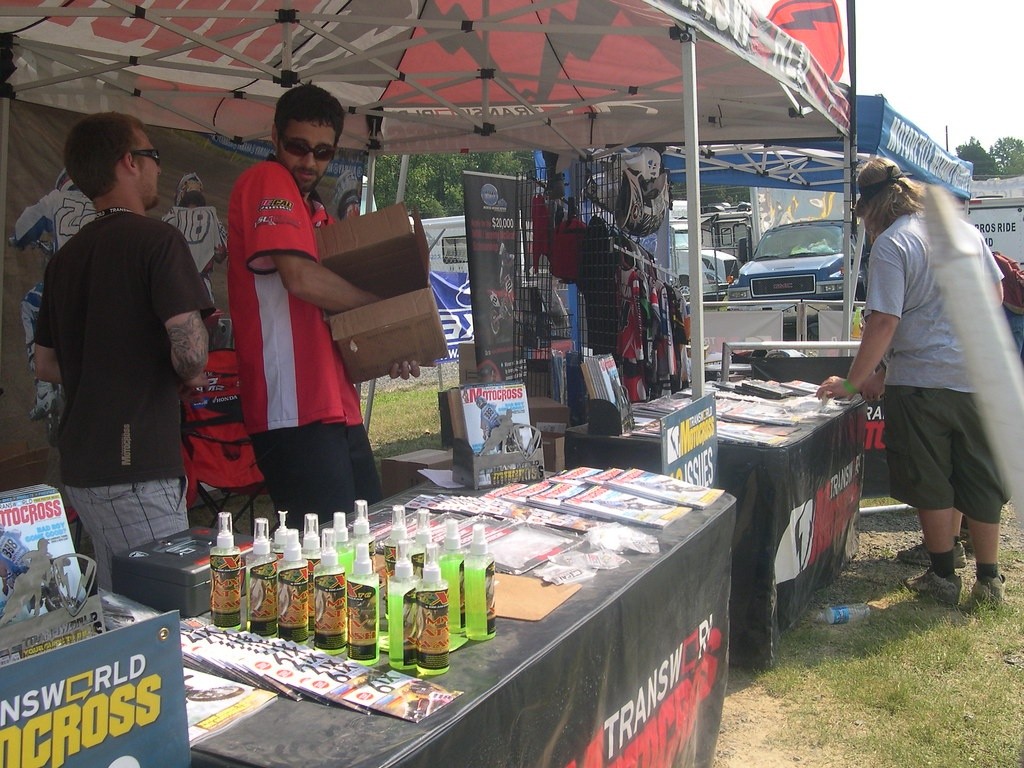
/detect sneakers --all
[897,542,968,569]
[905,571,961,608]
[950,576,1005,629]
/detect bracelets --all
[841,378,856,395]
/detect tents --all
[534,93,974,427]
[0,0,852,401]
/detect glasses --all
[277,131,335,161]
[130,148,161,167]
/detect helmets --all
[616,167,670,236]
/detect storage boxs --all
[458,342,480,385]
[451,408,545,491]
[527,395,570,437]
[541,431,565,472]
[0,536,107,668]
[380,447,453,495]
[586,366,635,435]
[110,523,255,618]
[314,201,448,385]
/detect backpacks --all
[992,249,1023,314]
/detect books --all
[347,466,726,575]
[631,379,821,447]
[178,615,464,724]
[0,484,93,665]
[584,353,628,411]
[447,384,537,486]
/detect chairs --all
[180,350,281,537]
[65,505,83,553]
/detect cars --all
[728,218,870,311]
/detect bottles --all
[817,602,871,626]
[209,500,497,675]
[850,308,862,338]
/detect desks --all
[564,393,870,668]
[188,470,738,768]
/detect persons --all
[992,252,1024,357]
[228,86,383,538]
[817,155,1004,609]
[34,112,216,593]
[862,368,973,568]
